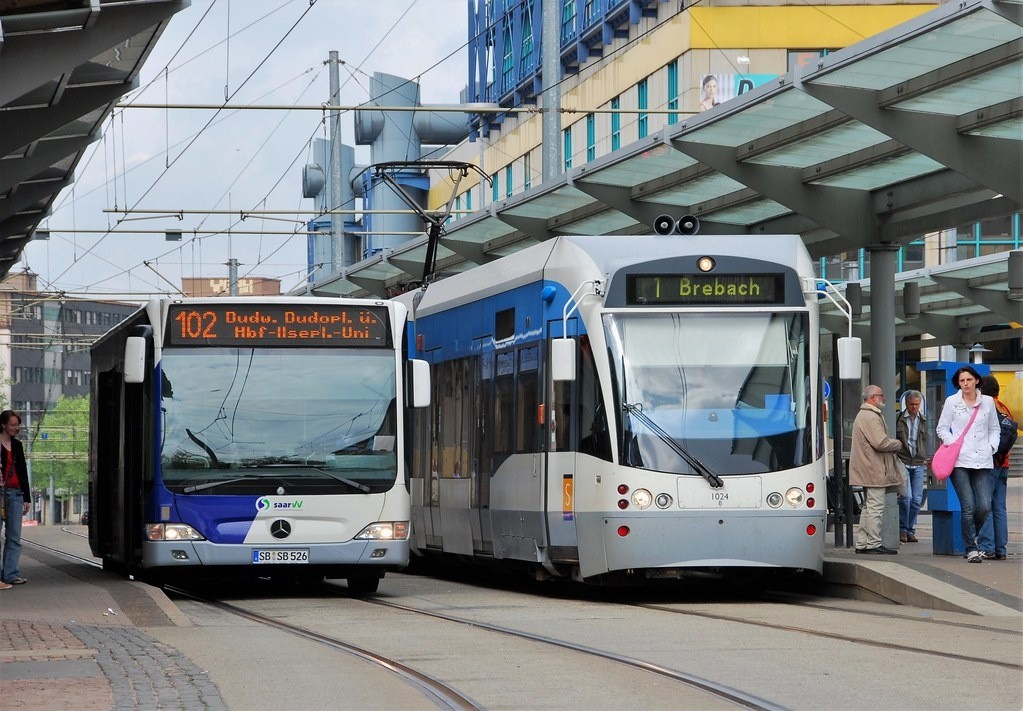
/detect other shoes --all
[995,554,1006,560]
[907,535,918,542]
[900,533,908,541]
[967,551,982,563]
[981,552,996,560]
[9,578,27,584]
[0,581,13,589]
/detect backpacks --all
[995,408,1018,464]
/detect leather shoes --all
[865,546,897,555]
[855,548,865,554]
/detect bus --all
[371,158,859,584]
[86,296,433,594]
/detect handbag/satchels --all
[0,490,7,520]
[931,406,979,480]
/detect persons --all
[848,385,903,554]
[935,366,1002,564]
[699,75,718,112]
[896,391,928,541]
[0,411,32,589]
[962,376,1017,561]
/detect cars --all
[82,511,89,524]
